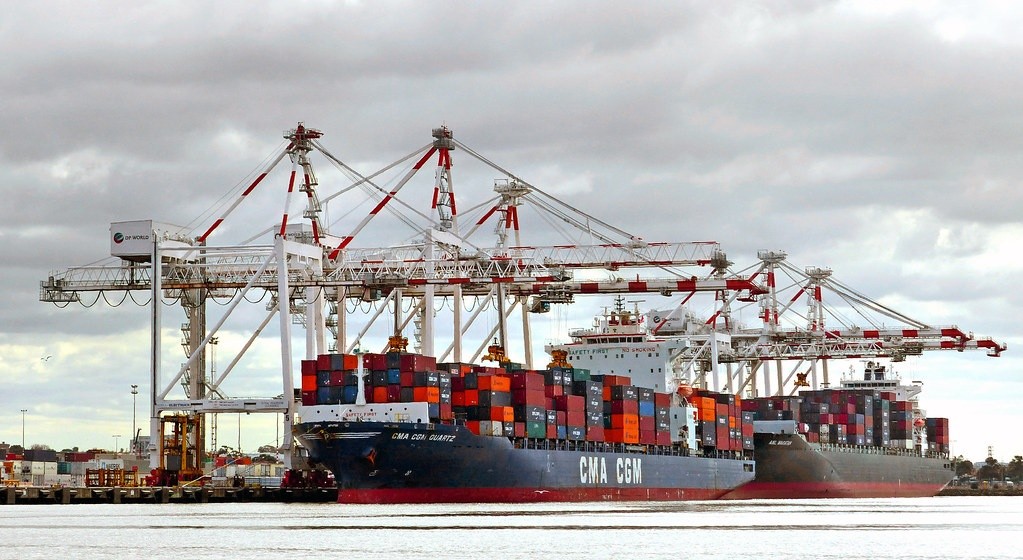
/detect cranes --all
[36,124,1005,456]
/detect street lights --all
[112,435,121,454]
[130,384,138,442]
[21,410,27,450]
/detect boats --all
[288,418,758,503]
[719,432,957,499]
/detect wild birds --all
[534,488,550,496]
[41,354,53,362]
